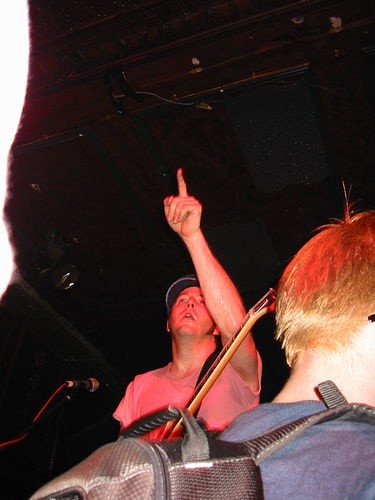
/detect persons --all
[112,168,263,445]
[216,179,375,500]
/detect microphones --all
[66,378,99,392]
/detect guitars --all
[137,287,277,446]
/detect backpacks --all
[26,403,375,500]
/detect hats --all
[165,274,200,317]
